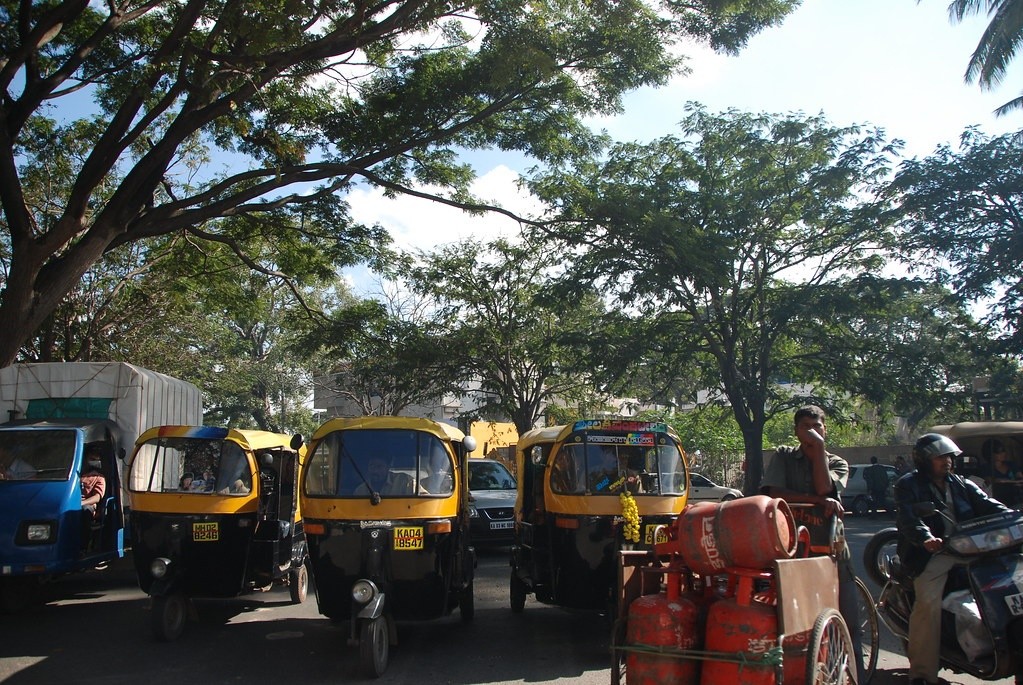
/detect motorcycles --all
[509,418,689,614]
[288,415,478,679]
[117,424,311,642]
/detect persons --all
[759,404,865,685]
[576,446,643,494]
[894,455,912,477]
[177,472,194,492]
[79,450,106,558]
[978,439,1023,479]
[353,448,430,495]
[218,453,262,518]
[0,430,37,479]
[863,456,896,519]
[893,433,1015,685]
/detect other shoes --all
[911,678,928,685]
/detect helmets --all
[913,433,957,471]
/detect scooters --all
[863,499,1023,685]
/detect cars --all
[838,462,905,517]
[465,459,521,550]
[926,420,1023,520]
[658,471,746,504]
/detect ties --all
[562,471,570,487]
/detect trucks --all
[0,360,202,592]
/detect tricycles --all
[607,502,878,685]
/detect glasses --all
[995,446,1006,454]
